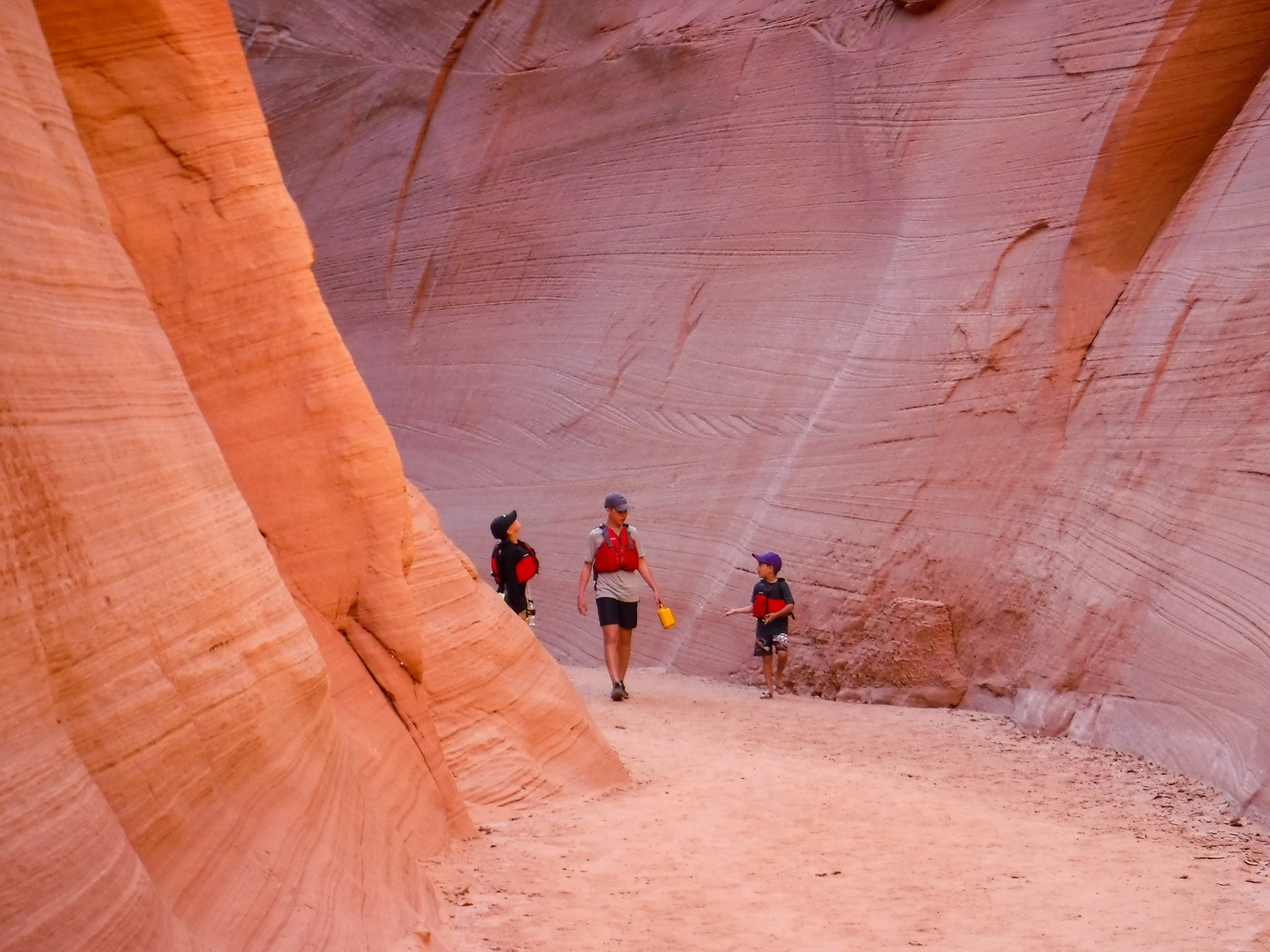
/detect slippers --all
[760,692,773,699]
[775,684,785,695]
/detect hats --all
[752,551,782,571]
[604,492,631,512]
[490,510,517,539]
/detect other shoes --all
[610,682,624,701]
[621,680,629,699]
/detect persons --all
[721,551,795,699]
[488,509,537,626]
[576,492,665,702]
